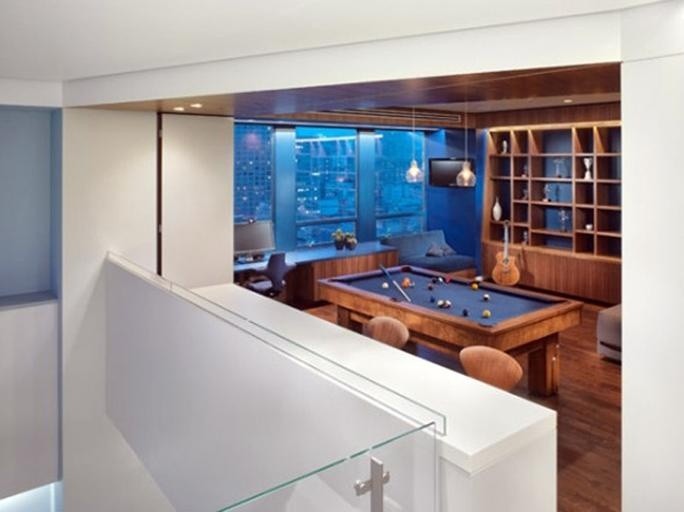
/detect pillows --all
[438,239,456,256]
[425,242,444,258]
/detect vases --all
[491,194,502,222]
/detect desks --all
[311,262,585,399]
[234,240,398,303]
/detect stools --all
[595,301,622,364]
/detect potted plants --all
[330,228,344,250]
[343,232,357,252]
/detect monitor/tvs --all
[429,158,476,188]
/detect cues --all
[377,263,411,303]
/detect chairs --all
[458,345,525,393]
[360,316,410,350]
[243,253,298,297]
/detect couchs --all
[384,230,479,278]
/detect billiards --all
[382,277,492,319]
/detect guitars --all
[492,220,519,286]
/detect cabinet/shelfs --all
[482,120,621,306]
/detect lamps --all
[455,88,477,188]
[406,105,424,183]
